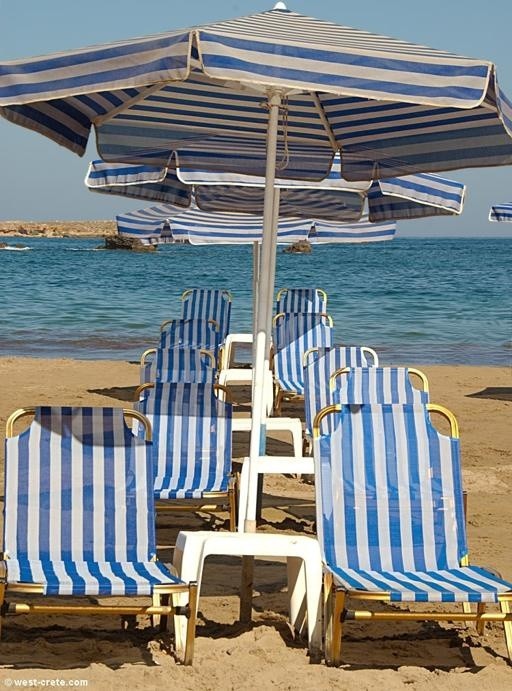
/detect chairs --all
[2,289,239,667]
[272,288,512,668]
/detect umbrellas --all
[81,150,472,465]
[0,0,512,525]
[484,201,511,226]
[106,198,400,362]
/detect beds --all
[165,331,324,664]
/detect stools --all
[165,331,324,664]
